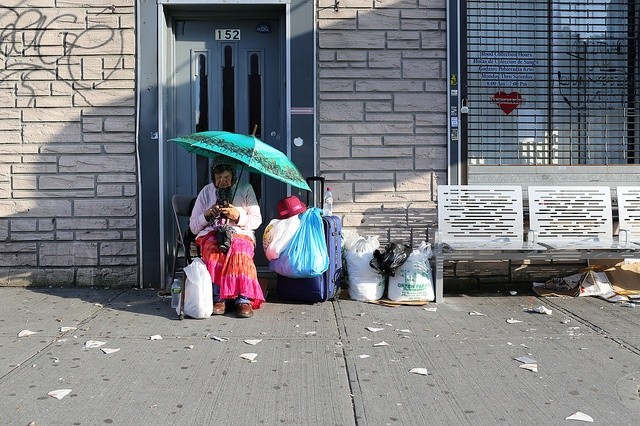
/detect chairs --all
[169,195,207,318]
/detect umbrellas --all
[166,124,313,225]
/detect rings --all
[209,208,213,214]
[214,204,221,208]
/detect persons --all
[189,155,266,318]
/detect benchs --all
[432,185,640,303]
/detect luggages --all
[277,177,341,302]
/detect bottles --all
[170,279,182,308]
[324,188,333,217]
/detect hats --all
[277,196,307,219]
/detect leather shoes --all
[213,301,225,314]
[236,303,254,318]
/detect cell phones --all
[217,203,230,214]
